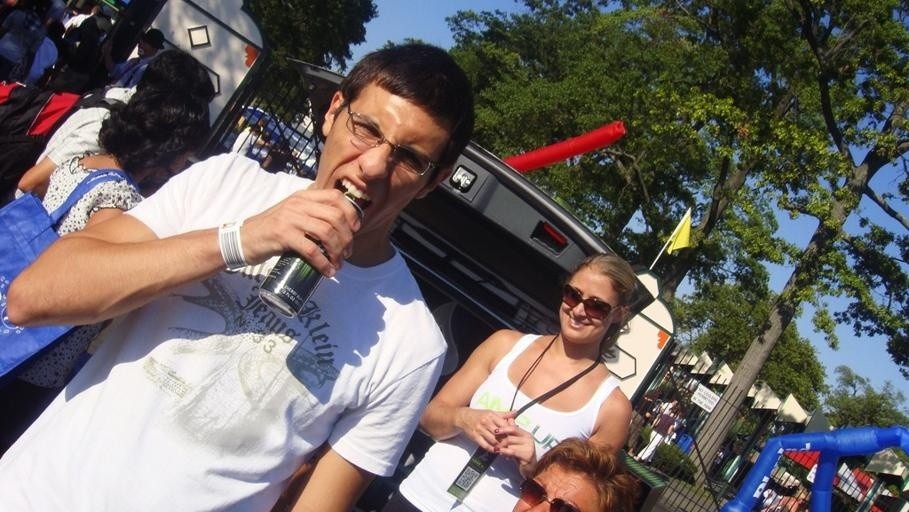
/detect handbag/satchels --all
[0,168,141,381]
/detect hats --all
[101,4,113,16]
[140,28,165,50]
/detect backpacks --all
[0,75,128,198]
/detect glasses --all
[562,283,621,322]
[519,478,580,512]
[346,99,445,176]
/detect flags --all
[667,213,692,256]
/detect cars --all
[238,101,303,177]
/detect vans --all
[286,53,653,437]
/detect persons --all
[0,0,681,512]
